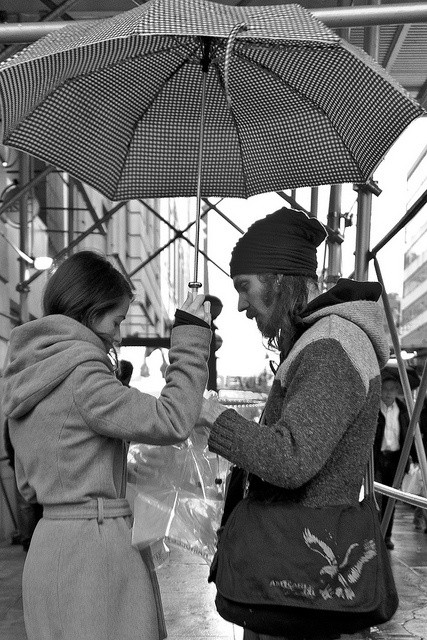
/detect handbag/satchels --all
[401,464,422,495]
[208,493,399,637]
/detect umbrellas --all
[1,2,427,298]
[381,359,421,394]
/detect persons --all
[369,366,414,550]
[116,358,134,388]
[192,206,402,639]
[0,247,214,640]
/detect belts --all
[381,451,391,455]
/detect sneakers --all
[384,537,394,549]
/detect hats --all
[230,206,328,278]
